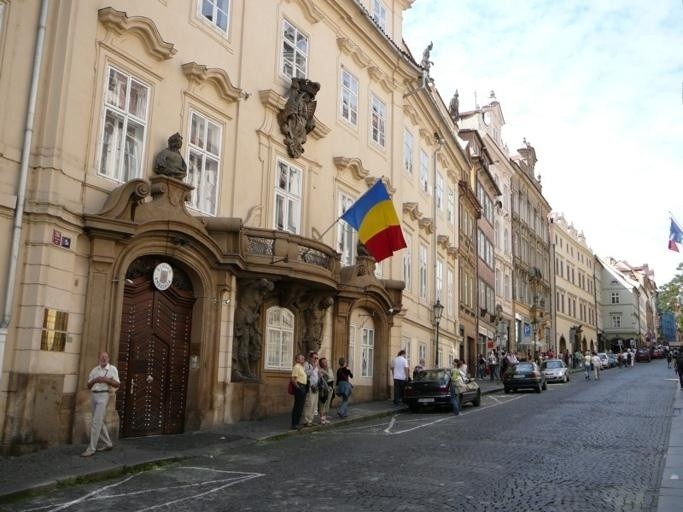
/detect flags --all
[667,217,683,253]
[343,181,407,264]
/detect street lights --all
[432,297,444,367]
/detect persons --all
[444,358,465,414]
[303,352,328,427]
[479,342,682,388]
[390,350,410,406]
[316,357,333,425]
[334,357,353,417]
[289,353,306,429]
[80,351,120,457]
[412,358,425,378]
[155,132,185,179]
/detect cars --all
[501,361,549,393]
[539,359,570,383]
[403,368,482,413]
[635,346,667,362]
[596,352,618,369]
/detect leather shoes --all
[80,451,92,456]
[96,447,112,451]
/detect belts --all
[92,390,108,393]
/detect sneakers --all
[320,419,325,425]
[324,420,330,424]
